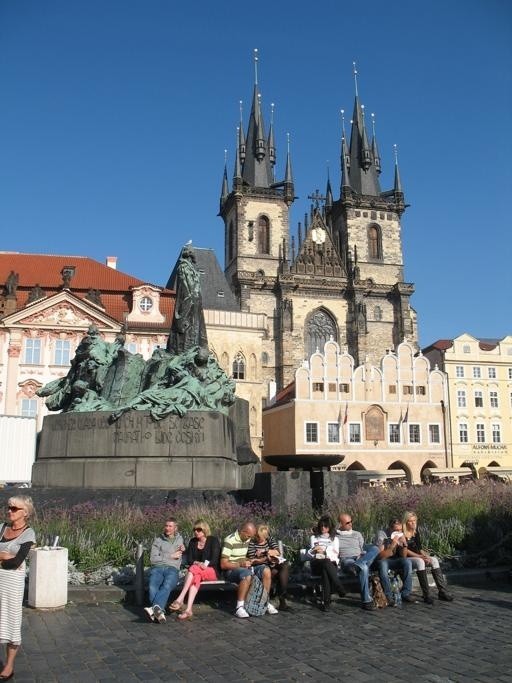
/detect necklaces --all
[11,524,27,531]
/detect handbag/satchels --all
[370,577,389,608]
[245,574,270,615]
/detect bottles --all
[391,578,401,604]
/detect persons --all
[143,518,188,625]
[247,523,295,611]
[36,329,239,418]
[0,493,37,682]
[168,520,222,622]
[308,515,346,612]
[372,518,416,607]
[219,522,279,618]
[335,513,380,611]
[167,245,208,354]
[401,510,453,605]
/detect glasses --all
[346,520,352,524]
[8,506,24,512]
[321,524,327,527]
[193,528,202,532]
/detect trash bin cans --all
[28,546,69,611]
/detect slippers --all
[169,600,184,610]
[177,610,190,619]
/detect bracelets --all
[403,543,408,547]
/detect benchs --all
[299,538,426,603]
[145,540,287,608]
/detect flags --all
[402,409,408,423]
[344,405,348,424]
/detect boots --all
[416,570,433,603]
[431,567,453,600]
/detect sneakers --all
[402,596,414,602]
[387,596,395,606]
[234,606,250,618]
[339,590,345,597]
[267,602,278,614]
[362,602,377,610]
[350,563,360,575]
[144,606,155,622]
[323,603,329,611]
[153,604,167,623]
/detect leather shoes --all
[0,671,14,682]
[278,605,294,611]
